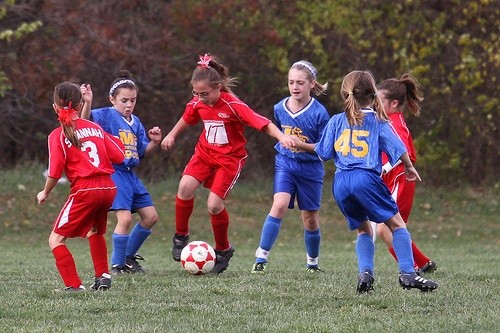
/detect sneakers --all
[90,276,111,291]
[355,269,375,294]
[399,274,438,291]
[305,266,326,276]
[53,285,86,293]
[211,246,235,274]
[415,261,438,277]
[172,234,189,261]
[251,259,268,275]
[111,255,147,275]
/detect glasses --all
[192,89,213,96]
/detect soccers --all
[180,241,216,275]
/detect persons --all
[375,74,437,278]
[160,54,296,274]
[37,82,125,294]
[80,70,162,276]
[251,60,331,275]
[313,70,439,293]
